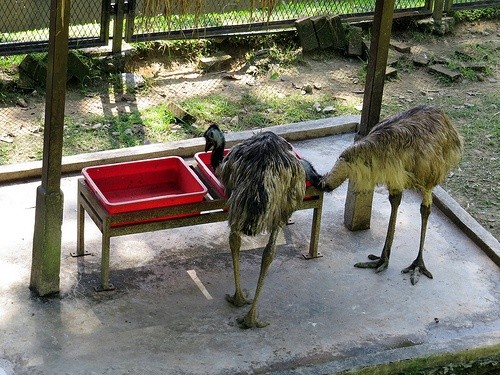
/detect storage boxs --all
[79,139,309,227]
